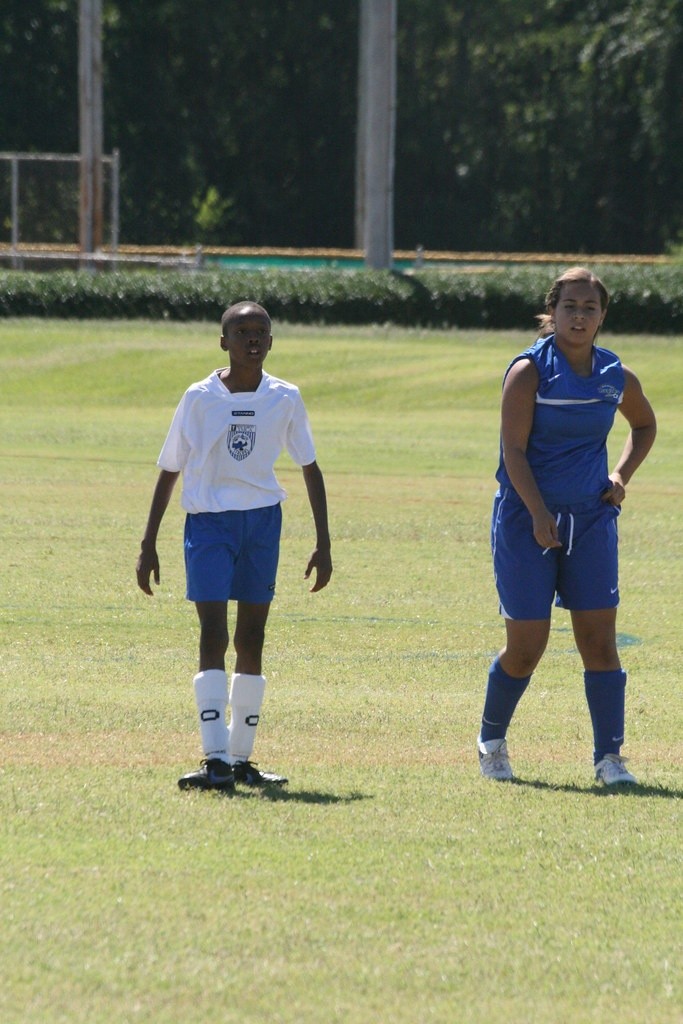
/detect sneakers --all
[230,760,289,788]
[178,757,235,793]
[594,753,636,788]
[476,732,515,781]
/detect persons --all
[132,299,335,797]
[473,265,660,792]
